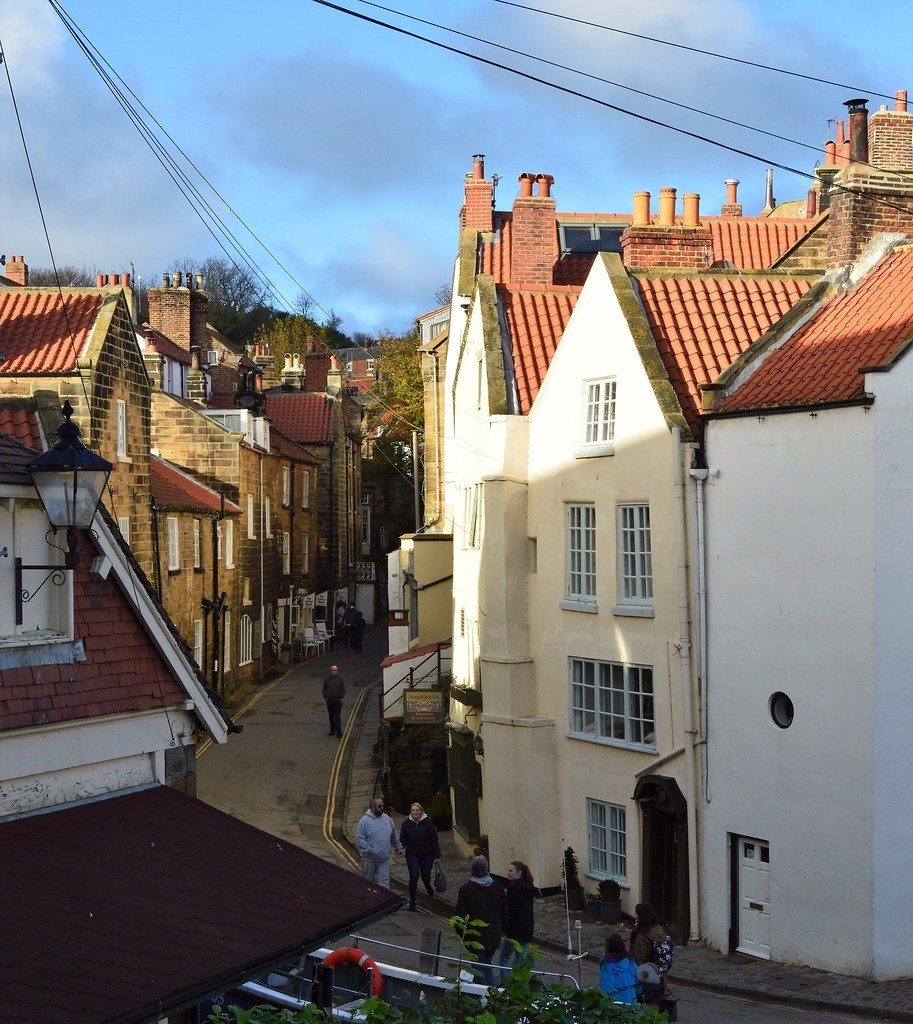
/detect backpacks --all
[648,936,674,974]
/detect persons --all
[628,903,667,996]
[598,933,643,1004]
[453,855,506,987]
[322,665,345,738]
[355,798,404,890]
[393,803,441,912]
[341,602,358,650]
[351,611,366,654]
[498,861,534,984]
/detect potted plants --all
[595,878,622,923]
[559,846,586,911]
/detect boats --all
[198,934,638,1024]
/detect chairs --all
[296,622,335,658]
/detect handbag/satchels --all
[433,860,448,893]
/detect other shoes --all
[336,732,343,737]
[329,731,334,736]
[427,888,433,895]
[408,903,416,912]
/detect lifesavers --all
[322,946,382,1012]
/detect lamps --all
[15,399,116,624]
